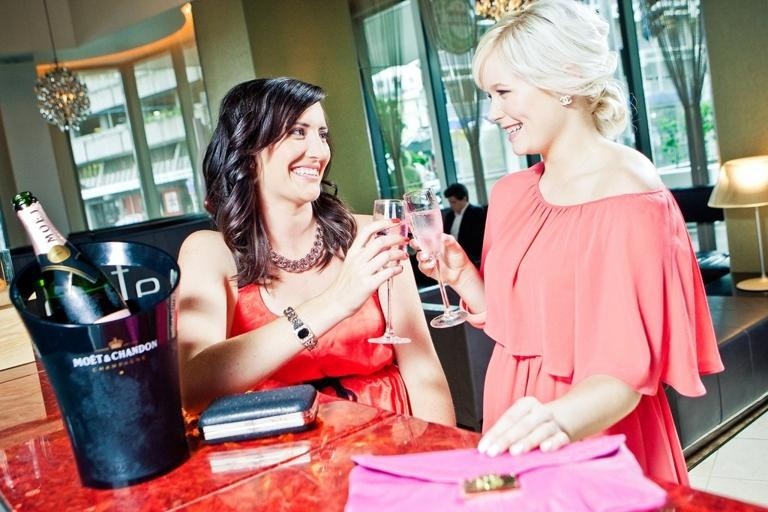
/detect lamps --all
[33,0,90,135]
[706,154,768,292]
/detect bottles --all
[10,191,131,325]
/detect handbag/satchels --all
[344,434,667,511]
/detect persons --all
[441,182,486,272]
[213,387,431,511]
[402,0,726,484]
[173,80,461,428]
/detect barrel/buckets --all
[10,239,189,489]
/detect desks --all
[1,360,766,512]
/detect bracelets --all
[285,306,318,351]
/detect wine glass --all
[368,198,412,345]
[402,187,469,329]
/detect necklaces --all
[268,219,328,275]
